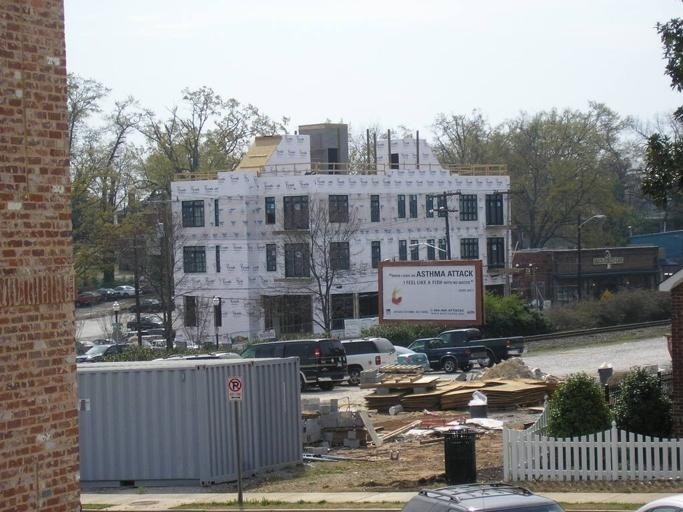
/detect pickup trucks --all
[427,328,529,370]
[405,338,488,373]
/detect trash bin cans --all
[471,405,487,419]
[598,368,613,384]
[441,428,480,486]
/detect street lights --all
[626,224,633,236]
[576,213,608,299]
[210,293,221,351]
[662,194,673,233]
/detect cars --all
[635,492,682,511]
[392,345,430,375]
[73,286,201,361]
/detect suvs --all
[149,353,244,360]
[238,336,349,393]
[336,337,398,385]
[400,482,564,511]
